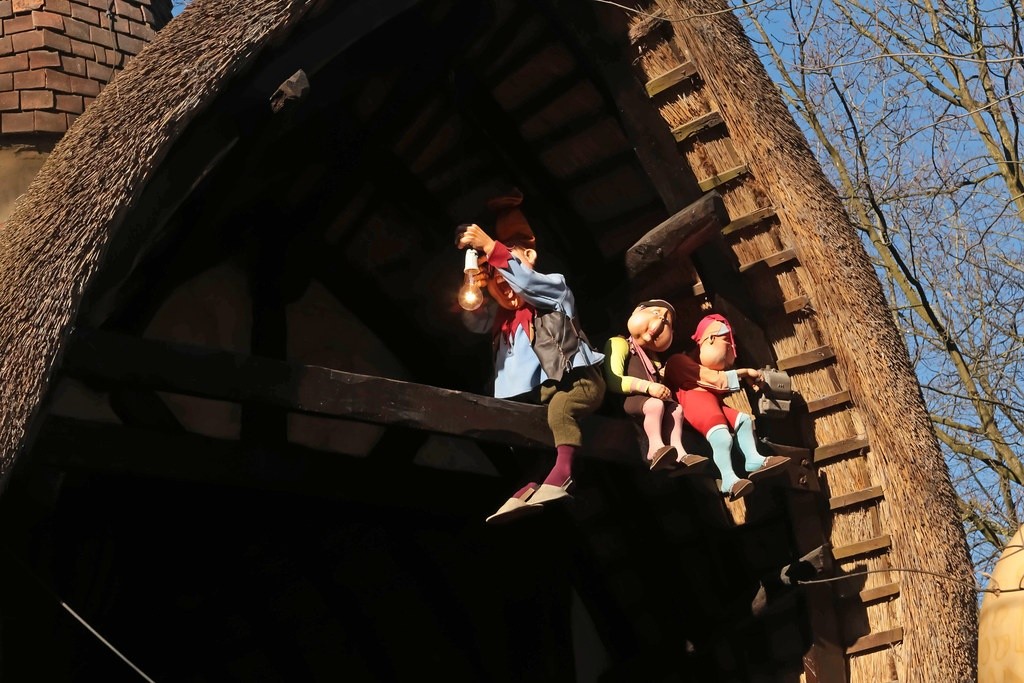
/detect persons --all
[436,184,609,527]
[663,300,793,504]
[601,297,710,480]
